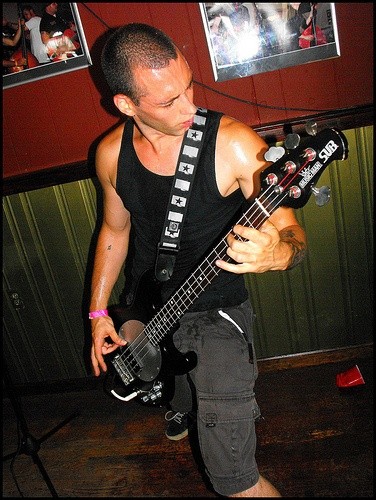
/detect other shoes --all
[166,413,189,441]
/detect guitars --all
[82,119,348,409]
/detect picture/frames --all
[198,2,341,82]
[2,2,94,89]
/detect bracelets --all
[88,309,109,320]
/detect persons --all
[206,2,316,65]
[2,2,80,76]
[88,23,307,497]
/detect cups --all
[335,364,366,388]
